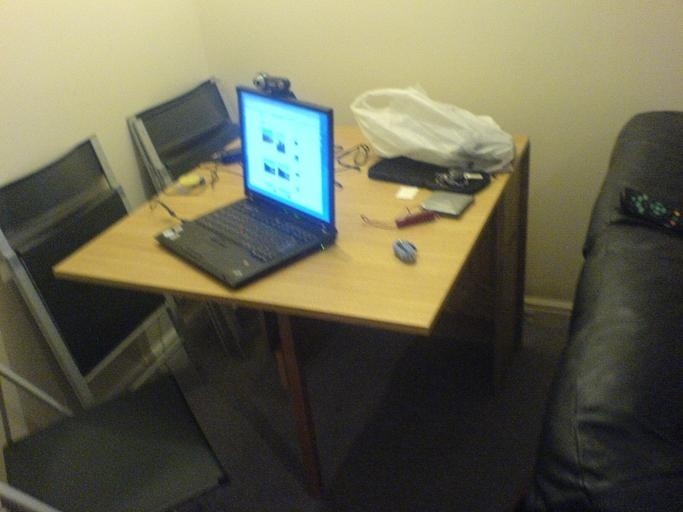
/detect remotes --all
[619,186,682,234]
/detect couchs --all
[532,110,682,511]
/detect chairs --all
[127,76,238,355]
[2,134,207,409]
[0,366,229,512]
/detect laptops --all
[153,86,338,289]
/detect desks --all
[53,123,531,511]
